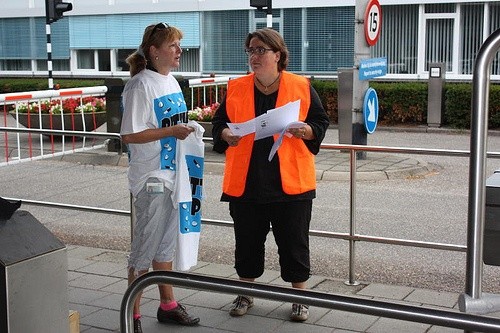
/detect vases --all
[9,111,106,138]
[197,121,213,137]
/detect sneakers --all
[229,294,254,315]
[157,303,200,326]
[291,303,309,321]
[133,318,142,333]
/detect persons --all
[208,29,331,318]
[120,24,207,333]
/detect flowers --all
[187,101,220,122]
[12,96,106,114]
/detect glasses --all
[148,22,170,39]
[245,47,275,56]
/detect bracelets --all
[301,127,307,137]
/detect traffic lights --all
[49,0,72,20]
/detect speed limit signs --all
[363,0,382,46]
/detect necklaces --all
[256,71,280,93]
